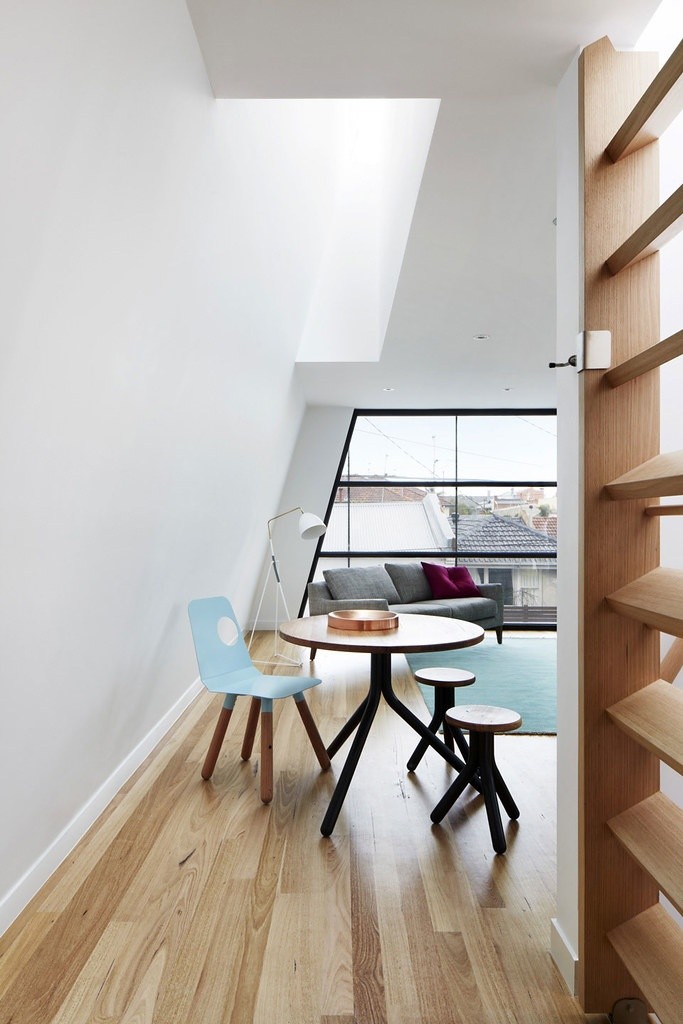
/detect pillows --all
[384,562,431,601]
[420,561,482,600]
[325,564,403,605]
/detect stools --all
[406,666,477,775]
[430,704,524,853]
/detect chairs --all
[188,596,333,803]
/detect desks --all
[280,614,485,838]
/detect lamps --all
[246,506,326,669]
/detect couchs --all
[308,564,504,661]
